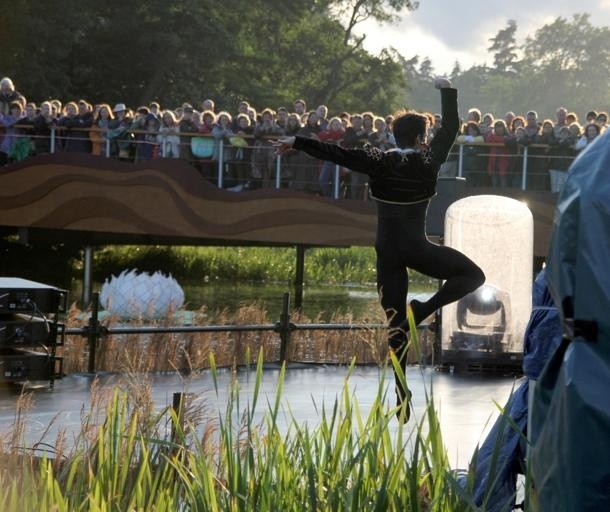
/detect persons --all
[268,78,486,427]
[0,77,609,192]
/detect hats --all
[112,104,129,114]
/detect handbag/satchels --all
[190,137,214,158]
